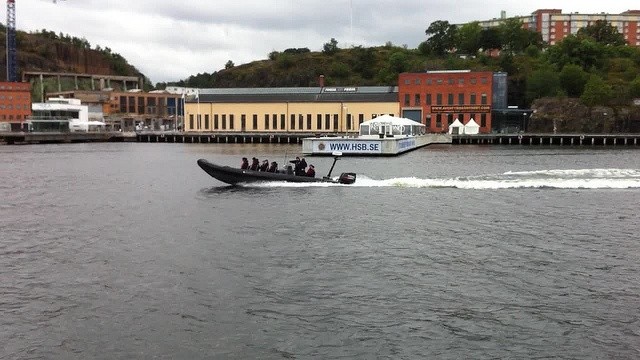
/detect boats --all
[196,152,358,189]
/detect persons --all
[241,157,315,177]
